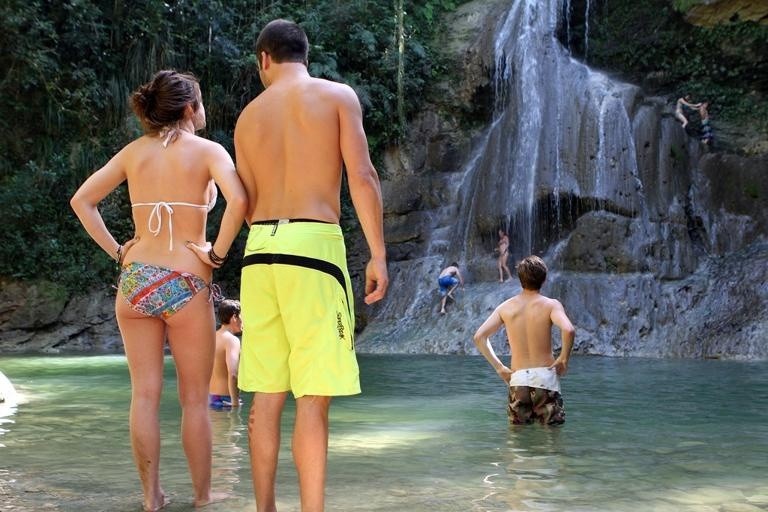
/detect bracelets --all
[208,249,228,266]
[115,244,123,263]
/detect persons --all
[68,67,250,512]
[698,98,713,145]
[492,228,512,283]
[436,261,464,314]
[207,298,243,414]
[674,89,704,128]
[473,254,575,428]
[232,19,389,512]
